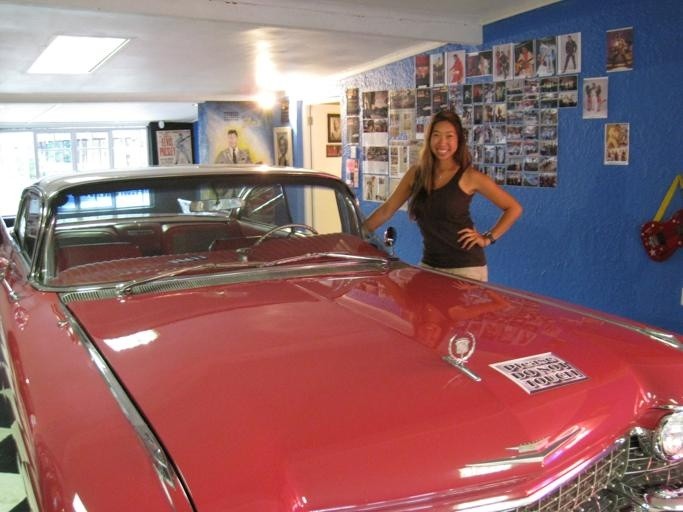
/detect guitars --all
[642,208,683,261]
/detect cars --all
[0,165,683,512]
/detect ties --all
[231,148,237,164]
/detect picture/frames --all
[273,127,293,167]
[328,114,342,142]
[326,145,342,157]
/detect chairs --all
[55,220,244,274]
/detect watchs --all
[482,231,497,244]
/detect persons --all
[215,129,253,164]
[332,119,341,140]
[277,137,288,165]
[365,112,523,283]
[173,133,192,164]
